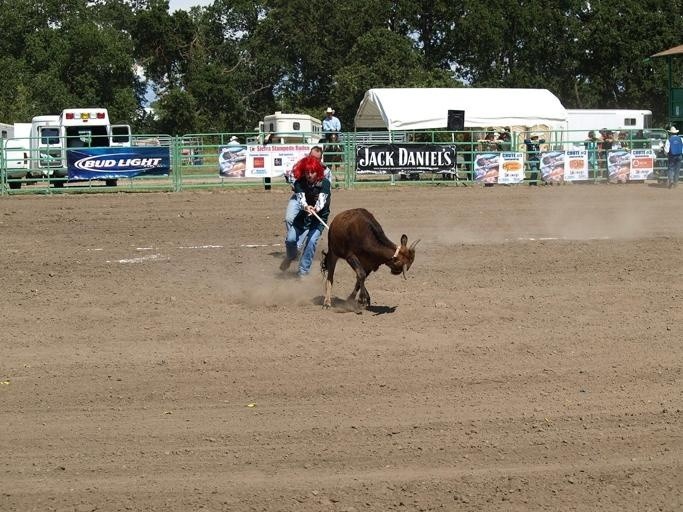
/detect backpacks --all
[668,136,683,156]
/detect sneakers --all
[280,258,291,272]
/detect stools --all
[2,167,27,188]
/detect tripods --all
[437,132,468,187]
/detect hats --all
[667,126,679,134]
[323,107,335,115]
[229,136,238,142]
[528,132,544,139]
[498,129,508,134]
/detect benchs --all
[54,169,118,188]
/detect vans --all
[1,107,133,189]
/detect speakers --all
[447,110,464,131]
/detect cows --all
[317,206,421,316]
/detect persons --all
[662,126,683,190]
[477,126,504,188]
[284,145,333,265]
[494,127,512,151]
[191,147,203,166]
[278,156,332,283]
[461,128,478,181]
[579,125,667,186]
[522,131,545,187]
[226,135,241,146]
[321,106,346,152]
[261,132,274,191]
